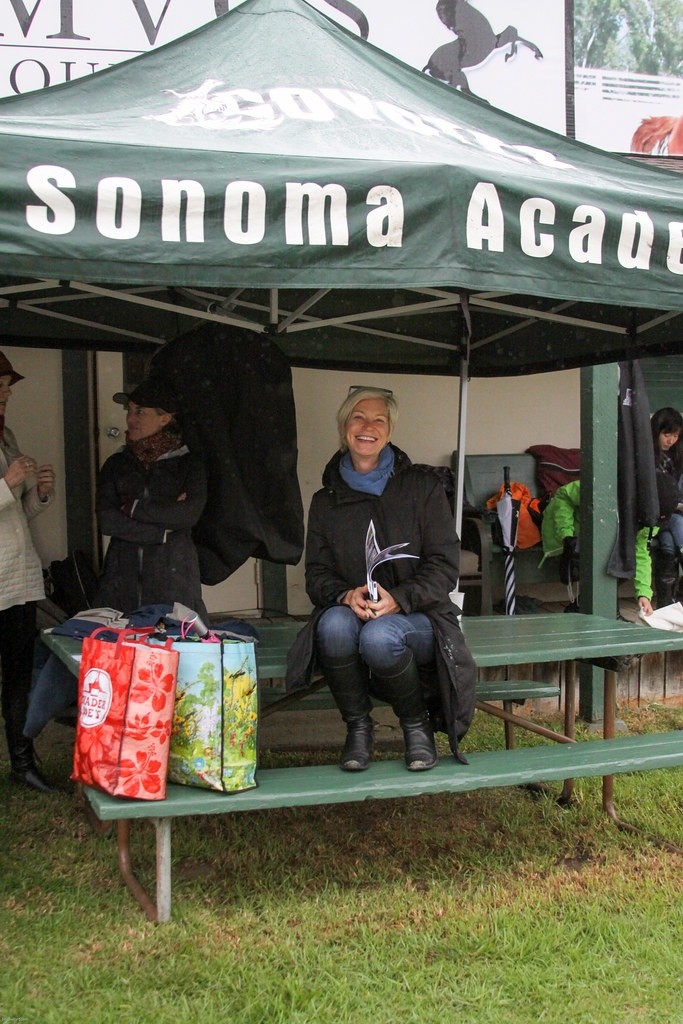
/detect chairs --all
[412,462,492,617]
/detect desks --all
[39,613,683,866]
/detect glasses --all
[348,386,393,397]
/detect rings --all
[25,462,30,468]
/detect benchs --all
[55,680,683,925]
[452,450,660,616]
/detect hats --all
[0,351,25,386]
[657,468,680,522]
[112,381,182,421]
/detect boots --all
[365,652,437,771]
[654,552,680,610]
[564,595,579,613]
[4,719,54,794]
[617,583,635,623]
[320,662,374,773]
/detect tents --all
[0,0,683,592]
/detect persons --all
[286,386,479,771]
[0,351,66,793]
[650,407,683,609]
[538,480,661,624]
[94,381,210,626]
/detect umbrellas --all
[497,466,519,616]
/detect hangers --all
[197,302,226,326]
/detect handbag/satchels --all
[69,627,257,801]
